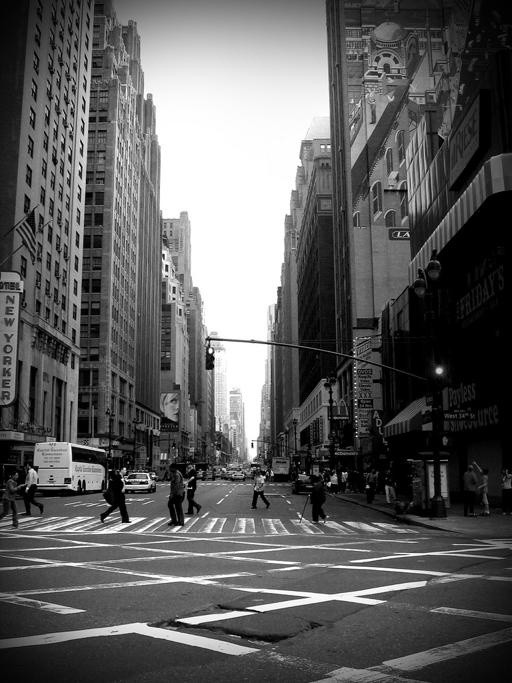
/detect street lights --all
[133,417,140,470]
[413,245,450,520]
[324,370,337,495]
[106,408,116,479]
[293,417,298,454]
[280,436,283,456]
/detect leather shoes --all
[101,513,104,522]
[175,522,184,526]
[197,505,201,513]
[168,521,177,526]
[22,512,31,515]
[122,519,131,522]
[39,504,44,514]
[185,511,193,515]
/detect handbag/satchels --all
[103,487,116,504]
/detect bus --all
[33,441,110,494]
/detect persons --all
[499,467,512,516]
[248,469,271,510]
[20,460,45,517]
[1,469,25,528]
[472,459,490,516]
[322,468,362,495]
[270,469,274,483]
[462,464,477,517]
[364,466,377,505]
[159,391,181,432]
[383,467,397,504]
[164,463,186,526]
[183,462,202,515]
[108,465,116,487]
[306,474,327,523]
[99,464,132,525]
[265,470,270,485]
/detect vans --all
[2,463,25,471]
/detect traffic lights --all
[205,346,215,369]
[429,355,447,389]
[259,454,261,458]
[172,441,176,449]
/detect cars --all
[291,473,320,495]
[193,460,261,480]
[107,468,160,493]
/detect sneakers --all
[266,503,270,508]
[324,515,330,522]
[250,506,257,508]
[312,520,319,523]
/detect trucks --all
[272,456,293,481]
